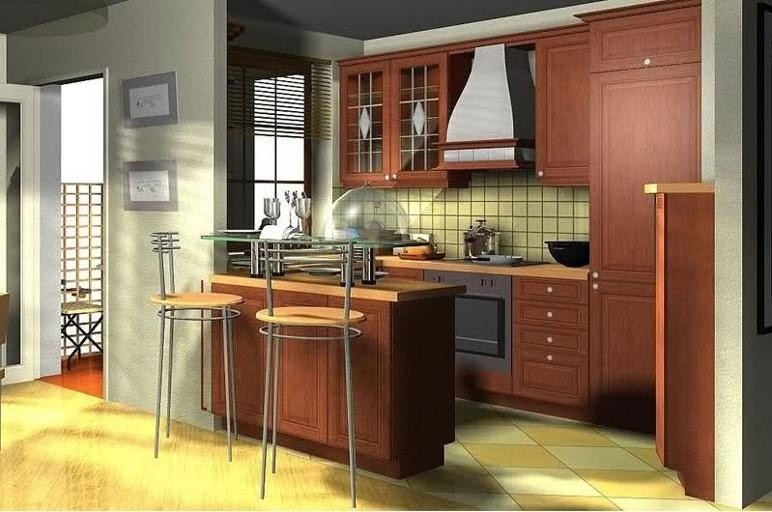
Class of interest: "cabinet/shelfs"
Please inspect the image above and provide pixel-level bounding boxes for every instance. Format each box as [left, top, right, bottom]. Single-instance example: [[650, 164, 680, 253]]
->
[[575, 3, 702, 434], [381, 267, 588, 423], [336, 49, 471, 188], [535, 29, 590, 187]]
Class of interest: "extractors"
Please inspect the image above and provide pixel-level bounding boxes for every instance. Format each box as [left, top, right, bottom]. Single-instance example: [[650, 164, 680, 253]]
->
[[442, 43, 537, 170]]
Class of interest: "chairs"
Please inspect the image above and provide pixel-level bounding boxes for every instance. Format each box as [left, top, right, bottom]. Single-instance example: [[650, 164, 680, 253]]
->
[[151, 232, 246, 462], [61, 279, 102, 371], [255, 241, 367, 509]]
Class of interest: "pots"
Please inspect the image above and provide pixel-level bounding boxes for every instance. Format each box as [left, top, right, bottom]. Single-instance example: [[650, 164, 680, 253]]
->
[[454, 254, 524, 266], [462, 219, 502, 259]]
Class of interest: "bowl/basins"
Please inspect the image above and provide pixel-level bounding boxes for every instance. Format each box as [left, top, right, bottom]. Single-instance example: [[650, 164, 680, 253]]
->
[[544, 241, 590, 267]]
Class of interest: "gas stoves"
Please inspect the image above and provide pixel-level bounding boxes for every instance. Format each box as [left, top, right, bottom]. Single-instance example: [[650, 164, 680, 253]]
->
[[441, 258, 552, 268]]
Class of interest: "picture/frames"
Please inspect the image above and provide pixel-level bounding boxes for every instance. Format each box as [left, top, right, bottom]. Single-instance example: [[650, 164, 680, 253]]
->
[[122, 71, 178, 130], [123, 160, 179, 211], [756, 2, 771, 333]]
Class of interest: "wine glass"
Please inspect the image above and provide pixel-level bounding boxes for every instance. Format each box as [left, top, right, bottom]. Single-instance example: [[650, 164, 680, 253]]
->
[[264, 189, 312, 241]]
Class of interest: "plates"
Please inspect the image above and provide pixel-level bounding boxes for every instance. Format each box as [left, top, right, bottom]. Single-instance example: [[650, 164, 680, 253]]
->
[[352, 270, 391, 277], [216, 229, 262, 233], [297, 267, 342, 276]]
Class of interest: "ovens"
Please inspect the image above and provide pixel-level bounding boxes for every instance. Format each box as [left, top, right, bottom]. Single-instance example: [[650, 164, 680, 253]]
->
[[423, 269, 513, 379]]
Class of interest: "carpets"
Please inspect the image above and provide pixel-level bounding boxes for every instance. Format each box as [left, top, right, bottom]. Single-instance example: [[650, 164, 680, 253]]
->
[[0, 379, 480, 511]]
